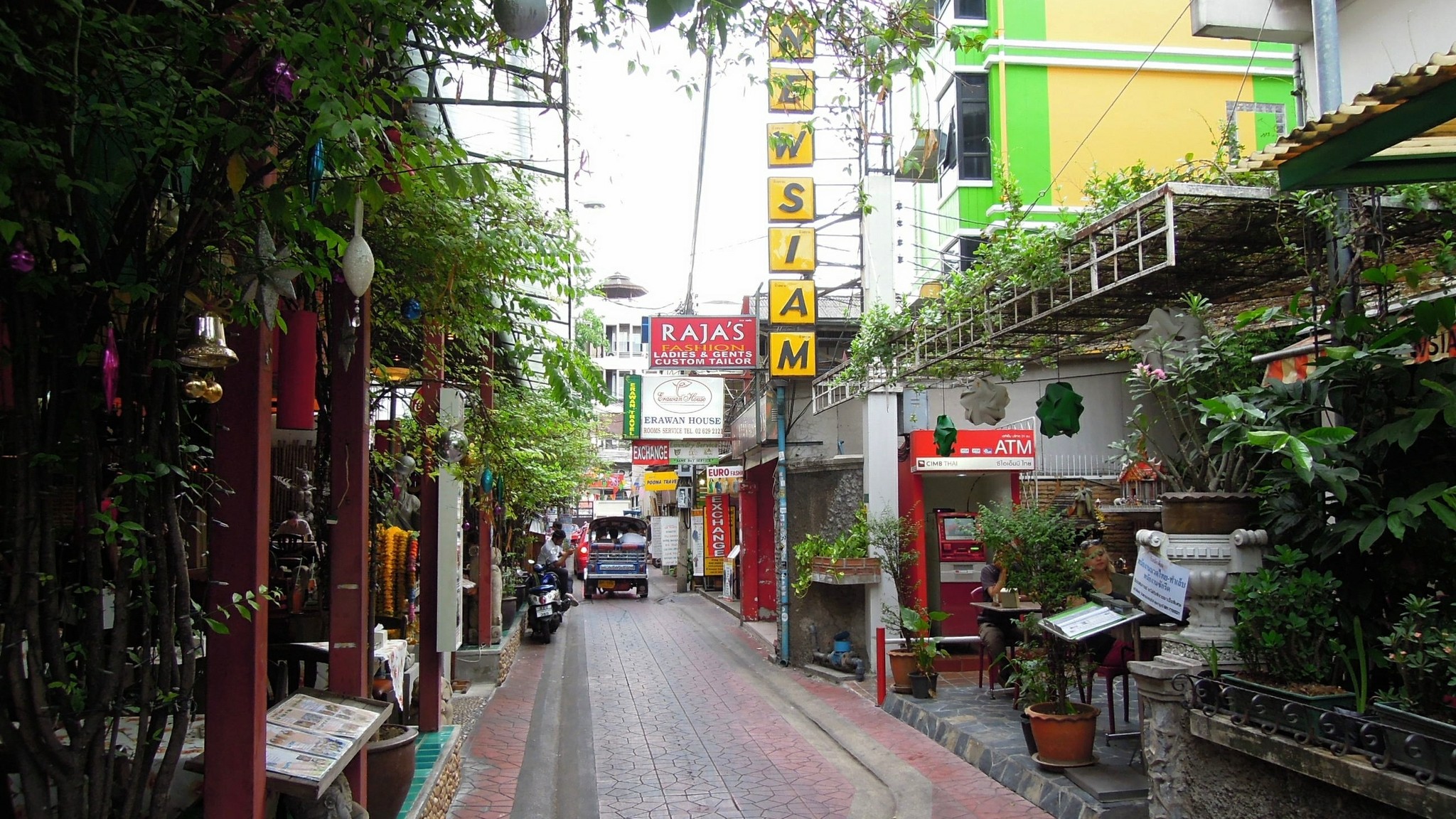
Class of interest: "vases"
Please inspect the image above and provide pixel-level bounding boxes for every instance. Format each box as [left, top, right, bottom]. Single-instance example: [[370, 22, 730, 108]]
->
[[1157, 493, 1267, 535], [366, 721, 419, 819]]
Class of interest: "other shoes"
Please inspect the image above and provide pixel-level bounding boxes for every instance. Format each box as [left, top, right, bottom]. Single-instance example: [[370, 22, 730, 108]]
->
[[565, 593, 578, 606]]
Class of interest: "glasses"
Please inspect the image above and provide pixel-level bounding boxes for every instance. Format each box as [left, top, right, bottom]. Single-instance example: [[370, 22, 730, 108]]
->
[[1080, 539, 1100, 549]]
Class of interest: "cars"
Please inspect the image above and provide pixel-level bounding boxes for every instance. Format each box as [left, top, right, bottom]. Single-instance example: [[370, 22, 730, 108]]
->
[[572, 528, 589, 579]]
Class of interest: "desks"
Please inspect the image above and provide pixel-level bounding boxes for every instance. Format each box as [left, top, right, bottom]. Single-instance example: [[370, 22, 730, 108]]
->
[[971, 602, 1063, 645], [287, 639, 408, 712], [271, 539, 328, 562]]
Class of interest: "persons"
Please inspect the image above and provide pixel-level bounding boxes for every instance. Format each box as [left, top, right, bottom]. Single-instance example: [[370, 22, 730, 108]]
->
[[709, 477, 714, 494], [375, 455, 422, 533], [724, 476, 731, 493], [977, 544, 1044, 704], [550, 521, 567, 570], [646, 514, 652, 563], [1064, 539, 1178, 677], [535, 528, 581, 607], [548, 526, 553, 533], [732, 476, 739, 493], [272, 509, 315, 549], [723, 565, 727, 598], [715, 476, 722, 495], [614, 523, 646, 545], [289, 463, 318, 523], [607, 529, 622, 544], [729, 571, 732, 601]]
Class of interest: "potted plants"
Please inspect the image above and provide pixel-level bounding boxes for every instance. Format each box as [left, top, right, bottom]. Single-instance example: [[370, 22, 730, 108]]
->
[[500, 528, 539, 630], [790, 493, 951, 698], [1219, 539, 1456, 787], [953, 497, 1106, 763]]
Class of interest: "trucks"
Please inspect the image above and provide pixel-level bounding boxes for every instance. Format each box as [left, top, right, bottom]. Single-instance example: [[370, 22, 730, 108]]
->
[[583, 516, 649, 599]]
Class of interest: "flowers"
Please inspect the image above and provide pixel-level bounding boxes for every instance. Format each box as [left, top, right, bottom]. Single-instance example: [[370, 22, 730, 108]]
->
[[1102, 288, 1291, 492]]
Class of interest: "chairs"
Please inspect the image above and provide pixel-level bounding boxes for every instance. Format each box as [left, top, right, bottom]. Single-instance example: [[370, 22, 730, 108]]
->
[[270, 532, 303, 623], [372, 615, 407, 722], [970, 587, 1132, 735]]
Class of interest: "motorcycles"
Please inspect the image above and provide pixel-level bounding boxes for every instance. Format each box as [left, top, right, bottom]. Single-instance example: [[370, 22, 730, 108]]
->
[[516, 555, 571, 645]]
